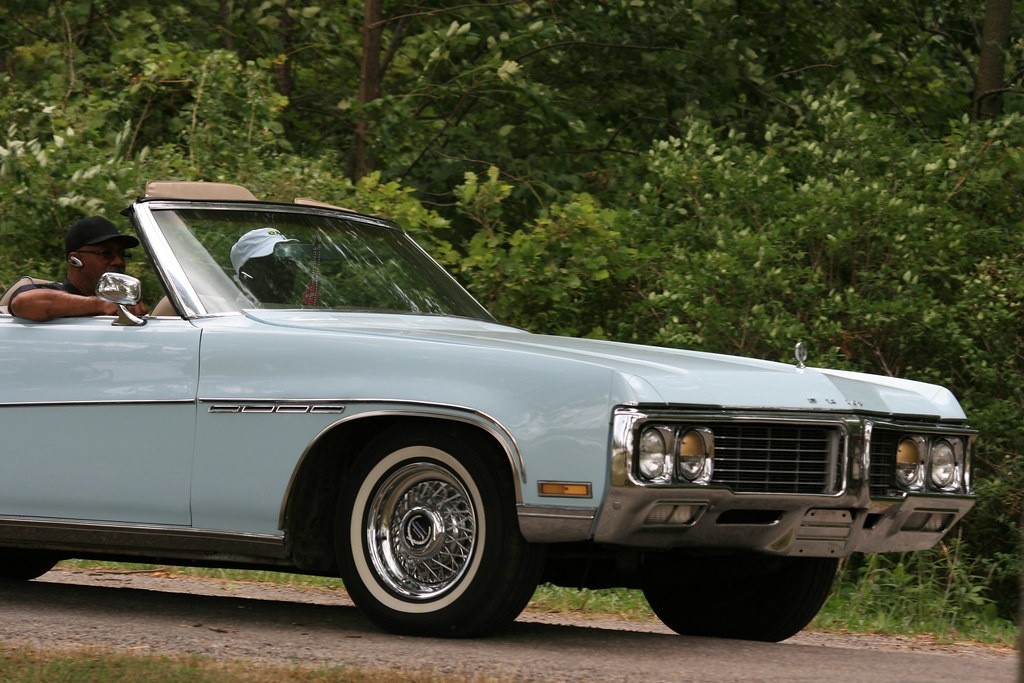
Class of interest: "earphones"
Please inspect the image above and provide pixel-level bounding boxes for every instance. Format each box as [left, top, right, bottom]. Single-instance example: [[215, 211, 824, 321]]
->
[[240, 271, 253, 281], [68, 256, 83, 267]]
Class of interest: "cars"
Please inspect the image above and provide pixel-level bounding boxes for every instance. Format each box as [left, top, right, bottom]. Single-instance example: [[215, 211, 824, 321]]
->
[[0, 182, 974, 643]]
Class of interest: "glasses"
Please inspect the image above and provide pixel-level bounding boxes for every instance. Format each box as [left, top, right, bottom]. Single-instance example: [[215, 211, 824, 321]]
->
[[74, 250, 133, 264]]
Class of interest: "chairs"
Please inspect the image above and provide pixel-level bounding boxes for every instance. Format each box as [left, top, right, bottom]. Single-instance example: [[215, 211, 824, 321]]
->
[[0, 276, 180, 317]]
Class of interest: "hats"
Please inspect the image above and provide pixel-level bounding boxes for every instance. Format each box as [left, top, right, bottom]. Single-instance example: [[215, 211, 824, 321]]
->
[[65, 216, 139, 263], [229, 227, 301, 277]]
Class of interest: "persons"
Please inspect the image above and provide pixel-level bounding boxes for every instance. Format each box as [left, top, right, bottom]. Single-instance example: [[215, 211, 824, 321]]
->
[[230, 227, 301, 304], [8, 217, 148, 322]]
